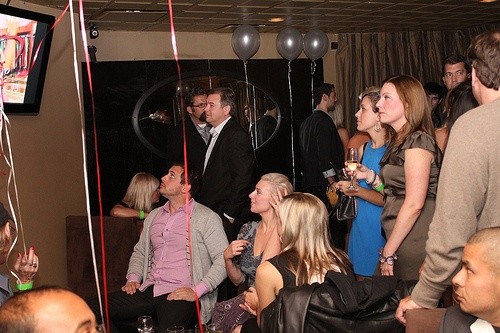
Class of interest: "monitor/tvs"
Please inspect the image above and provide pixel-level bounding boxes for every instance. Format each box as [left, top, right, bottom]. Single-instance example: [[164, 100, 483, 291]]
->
[[0, 4, 55, 115]]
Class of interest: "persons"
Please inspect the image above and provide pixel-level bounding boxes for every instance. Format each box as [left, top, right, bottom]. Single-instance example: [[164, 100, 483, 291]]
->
[[109, 172, 160, 218], [242, 104, 261, 132], [229, 191, 360, 333], [296, 81, 348, 253], [252, 99, 276, 149], [341, 73, 441, 282], [158, 110, 171, 125], [208, 172, 296, 333], [438, 226, 500, 333], [101, 162, 232, 333], [153, 26, 500, 254], [0, 284, 102, 333], [0, 203, 41, 308]]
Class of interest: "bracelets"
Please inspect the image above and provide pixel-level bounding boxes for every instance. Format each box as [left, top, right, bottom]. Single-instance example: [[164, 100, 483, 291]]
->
[[15, 279, 34, 291], [376, 182, 387, 193], [371, 176, 380, 186], [139, 210, 145, 220], [327, 179, 336, 189]]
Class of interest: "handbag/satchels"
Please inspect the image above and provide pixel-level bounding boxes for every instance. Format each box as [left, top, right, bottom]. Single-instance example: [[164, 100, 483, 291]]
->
[[329, 190, 357, 221]]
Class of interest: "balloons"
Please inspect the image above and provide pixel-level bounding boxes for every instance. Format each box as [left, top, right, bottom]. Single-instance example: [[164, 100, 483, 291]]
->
[[300, 28, 329, 62], [230, 26, 261, 63], [276, 26, 306, 63]]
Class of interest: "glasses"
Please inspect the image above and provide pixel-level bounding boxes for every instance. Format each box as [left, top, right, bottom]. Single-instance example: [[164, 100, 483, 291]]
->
[[9, 225, 16, 233], [192, 103, 208, 108]]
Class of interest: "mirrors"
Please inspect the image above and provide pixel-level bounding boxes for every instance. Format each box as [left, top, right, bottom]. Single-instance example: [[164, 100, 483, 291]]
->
[[132, 69, 284, 163]]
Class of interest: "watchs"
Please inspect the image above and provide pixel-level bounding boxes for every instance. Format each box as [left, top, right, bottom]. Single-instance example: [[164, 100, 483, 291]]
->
[[365, 172, 377, 186]]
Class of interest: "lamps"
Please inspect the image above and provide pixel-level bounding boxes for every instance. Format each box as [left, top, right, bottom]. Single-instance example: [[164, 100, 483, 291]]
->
[[90, 25, 99, 39]]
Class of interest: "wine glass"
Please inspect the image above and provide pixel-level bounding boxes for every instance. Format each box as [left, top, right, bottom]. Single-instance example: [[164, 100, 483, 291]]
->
[[345, 147, 358, 193]]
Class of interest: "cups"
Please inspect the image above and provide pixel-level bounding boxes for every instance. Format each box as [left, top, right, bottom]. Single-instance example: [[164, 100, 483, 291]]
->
[[195, 323, 223, 333], [166, 326, 184, 333], [137, 316, 154, 333]]
